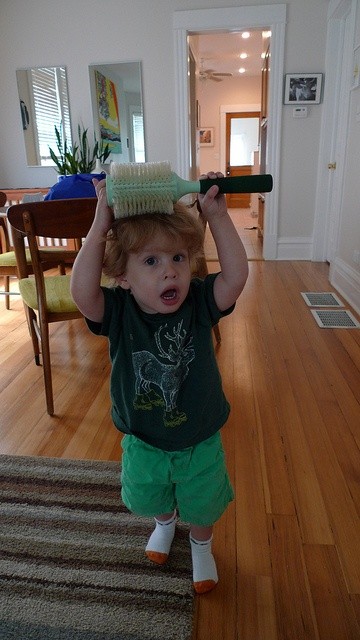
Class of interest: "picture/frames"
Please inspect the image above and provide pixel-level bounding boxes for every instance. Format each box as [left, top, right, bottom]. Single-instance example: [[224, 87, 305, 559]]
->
[[95, 70, 123, 155], [283, 73, 323, 106], [198, 127, 213, 147]]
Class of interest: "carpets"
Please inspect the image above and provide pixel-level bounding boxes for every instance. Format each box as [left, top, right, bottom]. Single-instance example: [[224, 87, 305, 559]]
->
[[0, 453, 195, 638], [311, 309, 358, 328], [301, 291, 344, 308]]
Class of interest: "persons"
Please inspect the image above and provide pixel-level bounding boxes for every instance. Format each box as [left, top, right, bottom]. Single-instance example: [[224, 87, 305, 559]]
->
[[70, 172, 249, 594]]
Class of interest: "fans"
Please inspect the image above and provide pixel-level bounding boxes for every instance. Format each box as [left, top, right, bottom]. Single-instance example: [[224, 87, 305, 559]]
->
[[200, 69, 232, 84]]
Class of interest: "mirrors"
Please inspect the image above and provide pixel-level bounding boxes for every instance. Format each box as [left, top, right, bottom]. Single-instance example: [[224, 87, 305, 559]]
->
[[88, 62, 147, 165], [15, 66, 75, 168]]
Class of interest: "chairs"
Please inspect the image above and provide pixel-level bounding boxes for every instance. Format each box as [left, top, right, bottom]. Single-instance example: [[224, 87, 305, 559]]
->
[[7, 198, 125, 414], [1, 214, 58, 311]]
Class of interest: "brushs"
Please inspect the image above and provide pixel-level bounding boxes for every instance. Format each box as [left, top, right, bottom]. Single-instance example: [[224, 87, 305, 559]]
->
[[105, 160, 275, 219]]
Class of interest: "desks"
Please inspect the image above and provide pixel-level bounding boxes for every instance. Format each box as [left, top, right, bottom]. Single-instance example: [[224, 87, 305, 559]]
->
[[1, 202, 223, 346]]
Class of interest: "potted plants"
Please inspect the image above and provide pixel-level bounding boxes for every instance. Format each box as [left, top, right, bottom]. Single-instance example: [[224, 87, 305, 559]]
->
[[79, 117, 108, 175]]
